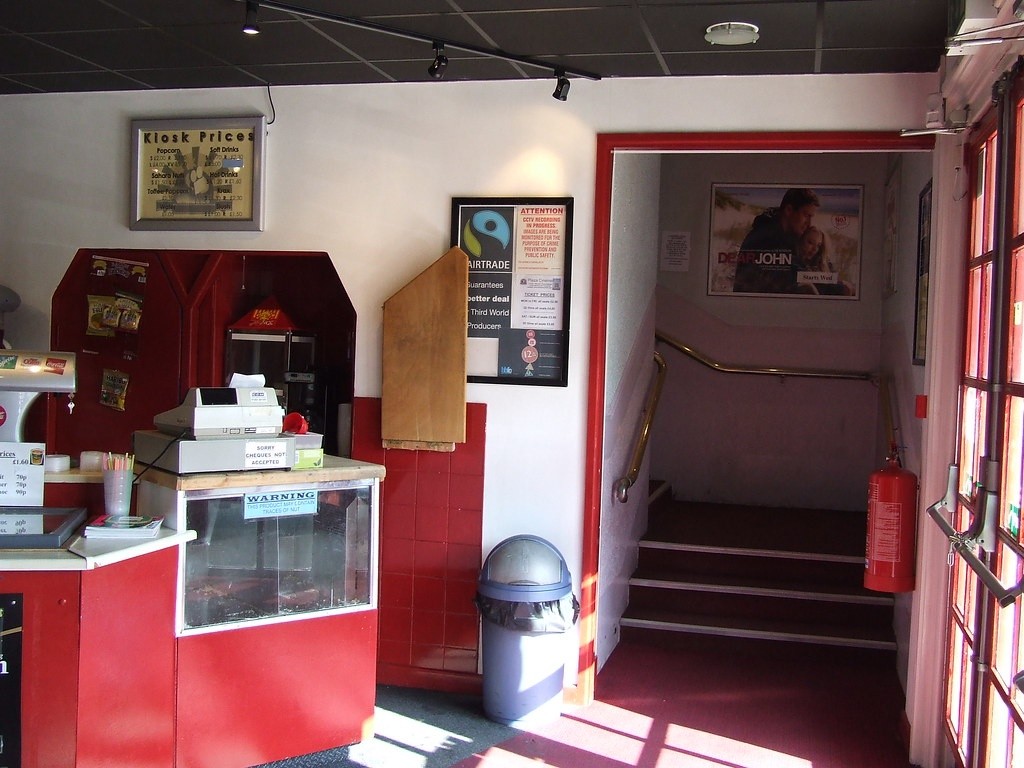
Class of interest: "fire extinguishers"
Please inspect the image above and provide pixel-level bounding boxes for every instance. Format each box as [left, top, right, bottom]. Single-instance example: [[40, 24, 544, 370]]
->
[[861, 437, 920, 593]]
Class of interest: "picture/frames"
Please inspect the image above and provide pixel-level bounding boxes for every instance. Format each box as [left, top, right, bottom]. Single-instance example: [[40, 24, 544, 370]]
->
[[450, 196, 574, 388], [129, 114, 266, 232], [706, 182, 865, 302]]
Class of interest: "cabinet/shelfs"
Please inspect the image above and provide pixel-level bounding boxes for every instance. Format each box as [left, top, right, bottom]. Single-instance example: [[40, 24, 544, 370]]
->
[[0, 523, 194, 768], [137, 454, 387, 768]]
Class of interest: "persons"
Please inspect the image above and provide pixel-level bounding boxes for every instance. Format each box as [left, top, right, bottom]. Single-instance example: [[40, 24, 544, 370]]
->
[[733, 188, 856, 296]]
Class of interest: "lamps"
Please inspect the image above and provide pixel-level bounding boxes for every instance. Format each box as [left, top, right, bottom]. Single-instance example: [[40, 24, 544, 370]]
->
[[241, 0, 602, 102]]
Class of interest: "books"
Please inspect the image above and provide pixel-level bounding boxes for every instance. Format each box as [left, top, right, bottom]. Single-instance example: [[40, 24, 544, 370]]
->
[[84, 514, 165, 539]]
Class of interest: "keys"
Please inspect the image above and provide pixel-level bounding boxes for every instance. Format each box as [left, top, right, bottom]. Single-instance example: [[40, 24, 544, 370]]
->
[[68, 392, 75, 414]]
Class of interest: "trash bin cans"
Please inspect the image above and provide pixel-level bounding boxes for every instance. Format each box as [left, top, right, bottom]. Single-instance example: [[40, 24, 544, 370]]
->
[[472, 533, 578, 731]]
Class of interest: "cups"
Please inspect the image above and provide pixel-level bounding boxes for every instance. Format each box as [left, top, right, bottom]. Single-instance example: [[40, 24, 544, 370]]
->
[[103, 469, 133, 516]]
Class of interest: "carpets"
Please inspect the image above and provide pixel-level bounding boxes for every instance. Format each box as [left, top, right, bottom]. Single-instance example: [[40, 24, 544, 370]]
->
[[349, 683, 529, 768]]
